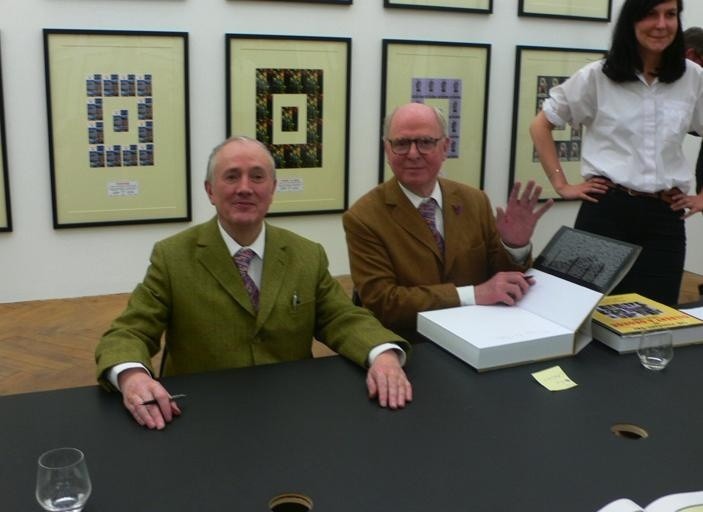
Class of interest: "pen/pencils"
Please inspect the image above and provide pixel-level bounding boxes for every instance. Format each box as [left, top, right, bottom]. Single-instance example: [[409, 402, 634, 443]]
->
[[292, 292, 297, 305], [139, 394, 188, 405], [523, 276, 533, 280]]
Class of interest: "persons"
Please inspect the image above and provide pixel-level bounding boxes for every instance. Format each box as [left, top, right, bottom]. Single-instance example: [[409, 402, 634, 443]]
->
[[93, 135, 414, 431], [343, 102, 555, 345], [683, 27, 703, 194], [528, 0, 703, 307]]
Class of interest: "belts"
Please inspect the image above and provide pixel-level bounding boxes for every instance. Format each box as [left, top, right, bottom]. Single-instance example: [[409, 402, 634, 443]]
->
[[606, 183, 664, 198]]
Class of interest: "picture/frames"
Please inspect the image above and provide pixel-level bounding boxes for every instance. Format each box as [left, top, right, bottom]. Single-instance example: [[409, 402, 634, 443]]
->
[[384, 0, 493, 13], [378, 38, 491, 191], [518, 0, 612, 22], [225, 33, 352, 216], [43, 28, 192, 229], [0, 52, 13, 232], [228, 0, 353, 4], [507, 45, 609, 203]]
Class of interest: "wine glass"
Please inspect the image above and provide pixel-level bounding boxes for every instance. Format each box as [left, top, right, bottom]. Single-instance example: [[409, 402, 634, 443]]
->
[[35, 447, 92, 512]]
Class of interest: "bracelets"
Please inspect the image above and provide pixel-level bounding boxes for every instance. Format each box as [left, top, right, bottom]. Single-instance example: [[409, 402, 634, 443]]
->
[[548, 168, 561, 179]]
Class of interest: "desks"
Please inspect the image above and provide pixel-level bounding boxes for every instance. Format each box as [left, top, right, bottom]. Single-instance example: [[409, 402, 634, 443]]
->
[[0, 300, 703, 511]]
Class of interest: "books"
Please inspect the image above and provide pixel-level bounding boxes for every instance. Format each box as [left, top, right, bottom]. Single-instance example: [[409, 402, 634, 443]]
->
[[415, 225, 645, 374], [591, 292, 702, 355]]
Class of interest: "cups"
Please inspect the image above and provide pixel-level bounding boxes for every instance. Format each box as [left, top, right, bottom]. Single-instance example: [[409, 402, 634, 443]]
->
[[637, 332, 674, 371]]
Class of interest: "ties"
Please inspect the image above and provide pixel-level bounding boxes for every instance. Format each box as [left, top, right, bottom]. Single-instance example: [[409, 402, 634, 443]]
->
[[232, 247, 261, 314], [417, 200, 446, 258]]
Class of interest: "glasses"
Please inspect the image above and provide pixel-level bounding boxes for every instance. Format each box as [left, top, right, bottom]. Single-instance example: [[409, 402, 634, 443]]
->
[[384, 136, 448, 157]]
